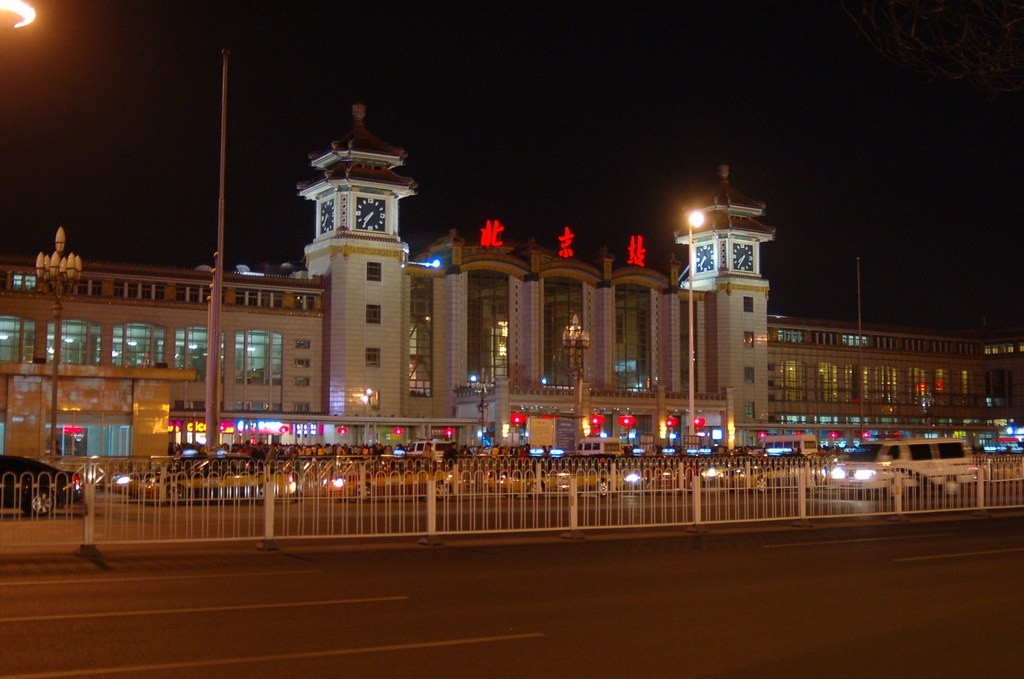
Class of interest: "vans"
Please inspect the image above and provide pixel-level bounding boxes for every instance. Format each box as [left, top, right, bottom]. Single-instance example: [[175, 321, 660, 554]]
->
[[405, 439, 453, 456], [576, 436, 622, 456], [830, 436, 979, 499]]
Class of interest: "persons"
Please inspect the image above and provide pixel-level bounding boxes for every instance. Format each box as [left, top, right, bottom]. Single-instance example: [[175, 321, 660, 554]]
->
[[165, 439, 843, 478]]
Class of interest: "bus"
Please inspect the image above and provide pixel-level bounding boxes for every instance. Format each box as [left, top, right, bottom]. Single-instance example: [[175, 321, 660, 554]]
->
[[758, 432, 818, 456]]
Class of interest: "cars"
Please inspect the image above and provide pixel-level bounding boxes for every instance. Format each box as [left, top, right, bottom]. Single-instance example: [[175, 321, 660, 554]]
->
[[0, 453, 82, 517], [113, 445, 830, 505]]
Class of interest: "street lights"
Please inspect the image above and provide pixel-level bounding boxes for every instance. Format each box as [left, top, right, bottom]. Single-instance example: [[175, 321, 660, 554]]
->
[[685, 209, 704, 436], [35, 225, 82, 457]]
